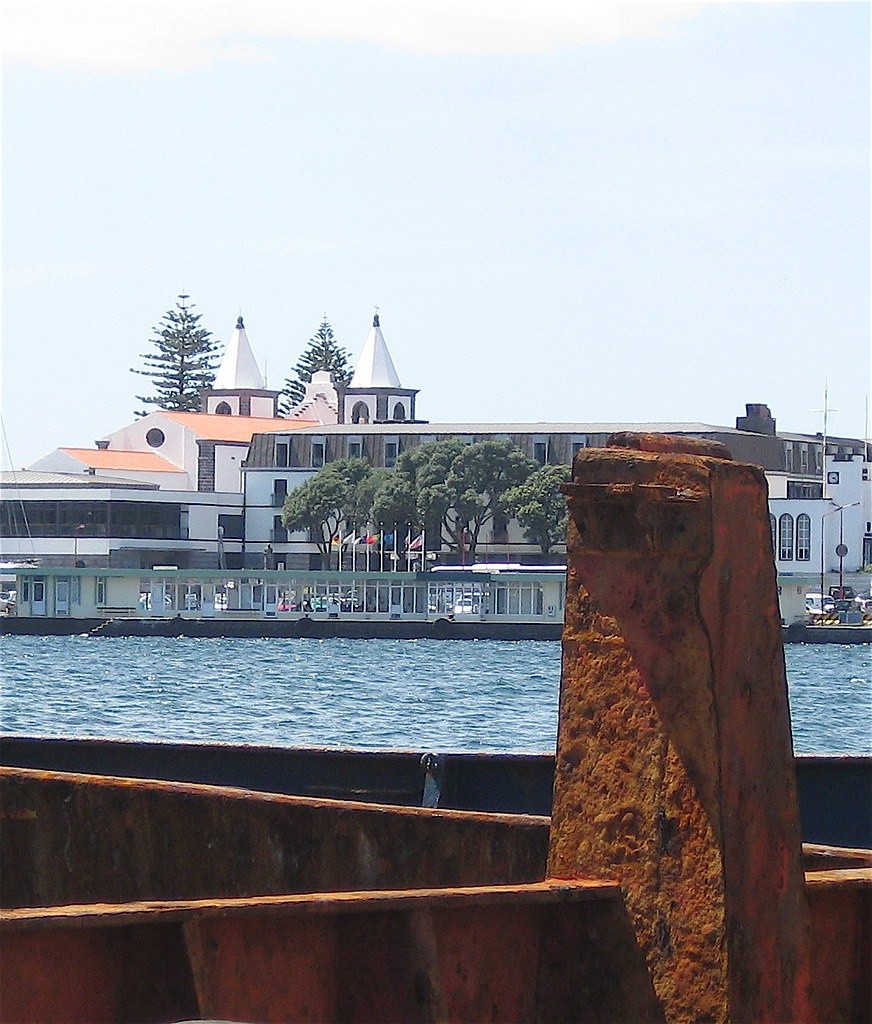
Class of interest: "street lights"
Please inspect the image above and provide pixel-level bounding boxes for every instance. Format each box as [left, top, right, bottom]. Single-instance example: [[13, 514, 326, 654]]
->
[[829, 500, 862, 626], [821, 507, 843, 612]]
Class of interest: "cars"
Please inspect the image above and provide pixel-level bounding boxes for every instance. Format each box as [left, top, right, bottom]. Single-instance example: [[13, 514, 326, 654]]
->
[[429, 592, 489, 614], [278, 590, 360, 612], [807, 584, 872, 624]]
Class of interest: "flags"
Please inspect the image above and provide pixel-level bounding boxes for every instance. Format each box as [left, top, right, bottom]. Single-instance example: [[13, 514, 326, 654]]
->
[[342, 533, 353, 544], [384, 534, 394, 545], [331, 532, 340, 546], [403, 536, 409, 548], [410, 536, 421, 550], [354, 535, 367, 547], [368, 533, 381, 545]]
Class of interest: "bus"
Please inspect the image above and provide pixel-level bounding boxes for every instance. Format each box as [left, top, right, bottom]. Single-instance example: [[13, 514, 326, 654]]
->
[[431, 563, 567, 590]]
